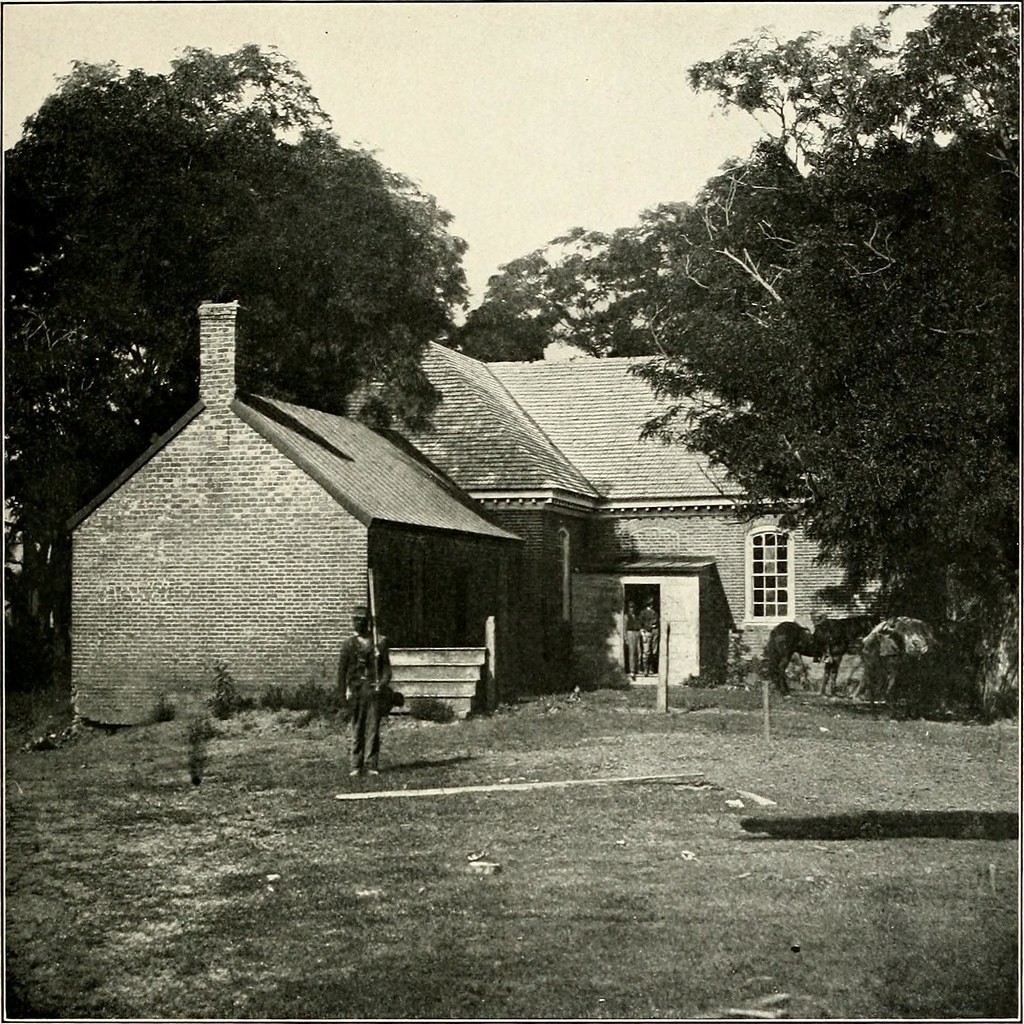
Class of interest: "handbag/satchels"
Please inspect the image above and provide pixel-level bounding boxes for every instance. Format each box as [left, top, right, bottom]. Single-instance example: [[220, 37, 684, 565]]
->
[[377, 684, 404, 717]]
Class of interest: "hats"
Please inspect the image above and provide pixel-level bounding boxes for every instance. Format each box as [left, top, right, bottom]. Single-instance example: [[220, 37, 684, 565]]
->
[[643, 596, 653, 604], [626, 601, 638, 609], [351, 607, 372, 617]]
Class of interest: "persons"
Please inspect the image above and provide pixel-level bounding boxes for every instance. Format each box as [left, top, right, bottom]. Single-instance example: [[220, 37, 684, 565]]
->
[[623, 597, 659, 678], [818, 619, 845, 696], [765, 590, 782, 616], [337, 607, 392, 777], [887, 645, 904, 720]]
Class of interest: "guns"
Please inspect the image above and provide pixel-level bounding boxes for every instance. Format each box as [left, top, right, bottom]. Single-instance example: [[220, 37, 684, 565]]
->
[[366, 569, 383, 692]]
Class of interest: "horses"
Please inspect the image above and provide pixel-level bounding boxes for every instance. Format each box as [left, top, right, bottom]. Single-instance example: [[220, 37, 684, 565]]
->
[[767, 611, 882, 702]]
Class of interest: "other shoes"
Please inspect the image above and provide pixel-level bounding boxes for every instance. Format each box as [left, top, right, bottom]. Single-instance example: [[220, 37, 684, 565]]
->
[[367, 769, 379, 776], [349, 769, 360, 777], [644, 668, 648, 677], [630, 672, 634, 679]]
